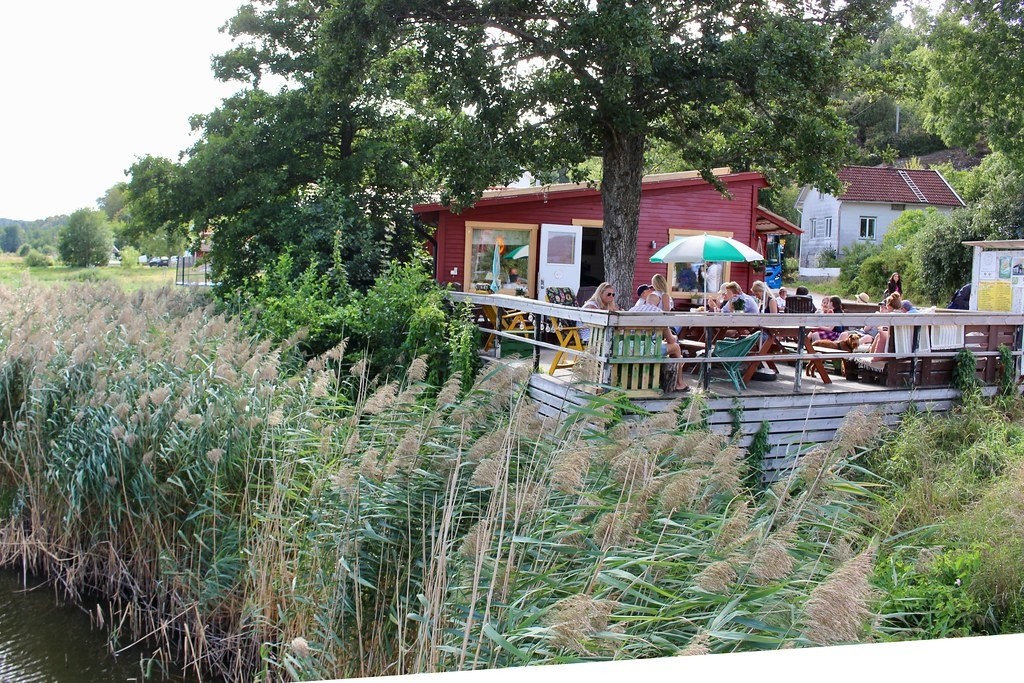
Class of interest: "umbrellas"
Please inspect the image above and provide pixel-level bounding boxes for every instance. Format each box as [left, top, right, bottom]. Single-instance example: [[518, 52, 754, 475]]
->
[[489, 242, 501, 294], [648, 233, 765, 334], [504, 244, 529, 259]]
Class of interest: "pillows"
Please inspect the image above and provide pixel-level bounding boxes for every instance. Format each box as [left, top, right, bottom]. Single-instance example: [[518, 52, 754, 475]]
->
[[870, 331, 887, 362]]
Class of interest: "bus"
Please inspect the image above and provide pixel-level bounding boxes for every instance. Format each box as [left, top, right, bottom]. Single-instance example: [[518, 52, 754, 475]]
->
[[764, 233, 788, 289]]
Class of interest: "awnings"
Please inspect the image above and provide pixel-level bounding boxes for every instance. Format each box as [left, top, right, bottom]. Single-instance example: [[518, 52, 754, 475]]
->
[[756, 204, 805, 236]]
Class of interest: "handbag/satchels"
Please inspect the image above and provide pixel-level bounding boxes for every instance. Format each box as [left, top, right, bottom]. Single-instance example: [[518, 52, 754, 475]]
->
[[884, 290, 891, 299]]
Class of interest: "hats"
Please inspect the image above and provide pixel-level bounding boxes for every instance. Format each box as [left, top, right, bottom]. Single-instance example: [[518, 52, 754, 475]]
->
[[636, 283, 653, 296], [854, 293, 870, 305], [718, 282, 731, 293], [878, 299, 887, 306], [901, 300, 912, 311]]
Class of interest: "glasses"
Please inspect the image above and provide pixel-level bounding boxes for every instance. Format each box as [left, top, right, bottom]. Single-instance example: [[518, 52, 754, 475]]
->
[[604, 292, 616, 297]]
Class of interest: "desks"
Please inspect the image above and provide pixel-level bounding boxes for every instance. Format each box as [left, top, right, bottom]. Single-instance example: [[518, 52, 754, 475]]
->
[[679, 339, 716, 374], [680, 302, 709, 307], [779, 342, 848, 378]]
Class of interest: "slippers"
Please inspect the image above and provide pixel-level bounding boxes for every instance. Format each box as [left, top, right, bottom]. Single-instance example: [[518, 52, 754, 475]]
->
[[676, 385, 691, 392]]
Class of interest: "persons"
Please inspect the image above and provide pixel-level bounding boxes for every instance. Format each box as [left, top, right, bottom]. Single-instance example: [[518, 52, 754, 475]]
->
[[796, 286, 816, 341], [679, 263, 698, 293], [887, 272, 903, 295], [626, 274, 687, 390], [510, 269, 520, 295], [709, 278, 778, 371], [577, 282, 619, 382], [698, 262, 722, 292], [837, 292, 916, 362], [947, 283, 971, 310], [807, 297, 845, 342], [775, 287, 794, 341]]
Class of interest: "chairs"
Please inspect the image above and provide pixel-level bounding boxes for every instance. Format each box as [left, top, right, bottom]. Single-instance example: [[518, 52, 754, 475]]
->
[[697, 330, 762, 394], [783, 296, 817, 313], [543, 287, 587, 375], [474, 283, 534, 352]]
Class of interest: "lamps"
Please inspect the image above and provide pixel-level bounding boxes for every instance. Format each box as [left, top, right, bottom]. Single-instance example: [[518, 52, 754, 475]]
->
[[652, 240, 656, 248]]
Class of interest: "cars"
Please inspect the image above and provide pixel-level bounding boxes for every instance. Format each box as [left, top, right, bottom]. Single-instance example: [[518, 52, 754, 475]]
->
[[149, 256, 169, 267]]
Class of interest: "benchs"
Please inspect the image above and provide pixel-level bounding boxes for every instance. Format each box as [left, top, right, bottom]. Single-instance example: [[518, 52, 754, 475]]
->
[[846, 325, 1017, 386], [842, 302, 880, 315]]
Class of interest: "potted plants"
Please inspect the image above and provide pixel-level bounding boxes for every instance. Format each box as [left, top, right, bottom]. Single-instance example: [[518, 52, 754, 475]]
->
[[753, 260, 767, 273], [690, 292, 700, 304]]
